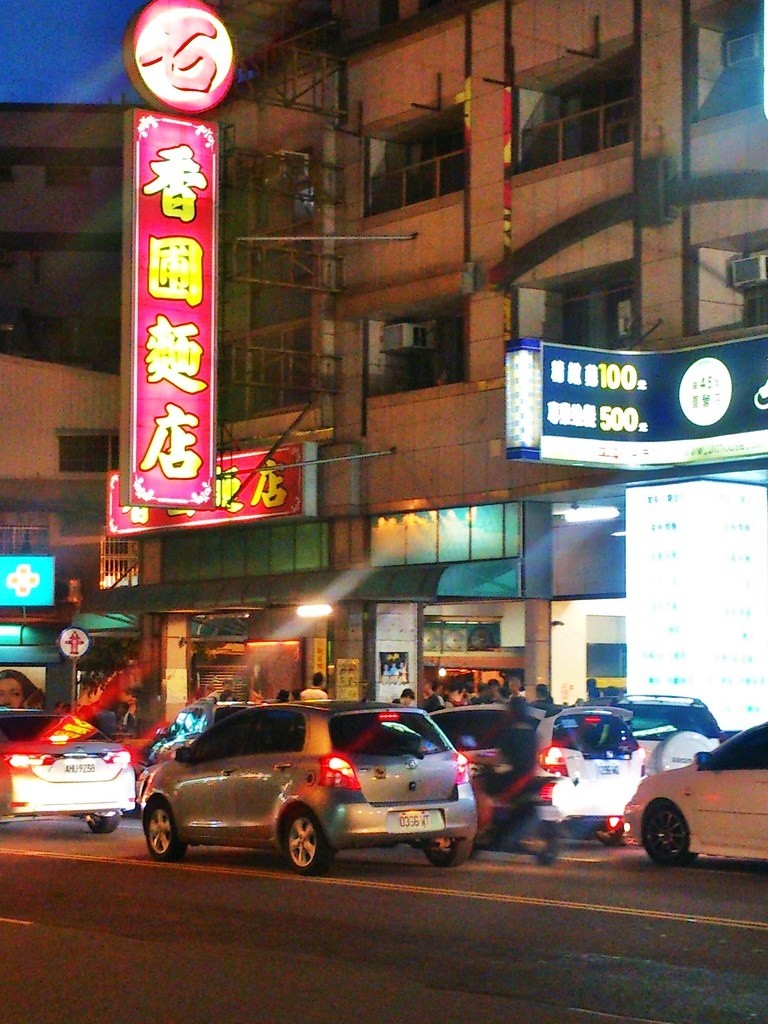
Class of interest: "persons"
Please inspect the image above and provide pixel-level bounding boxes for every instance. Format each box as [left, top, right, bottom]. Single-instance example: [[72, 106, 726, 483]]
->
[[0, 669, 45, 709], [191, 653, 198, 688], [587, 678, 600, 699], [115, 702, 134, 732], [392, 673, 553, 716], [206, 666, 328, 703], [340, 669, 353, 686], [55, 701, 81, 716], [382, 661, 405, 677]]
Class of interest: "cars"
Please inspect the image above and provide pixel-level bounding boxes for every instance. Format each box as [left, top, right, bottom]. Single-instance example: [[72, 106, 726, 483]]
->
[[139, 699, 478, 876], [621, 717, 768, 869]]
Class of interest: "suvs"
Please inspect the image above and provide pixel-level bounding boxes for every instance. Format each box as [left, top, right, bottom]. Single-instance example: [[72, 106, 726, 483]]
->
[[404, 694, 724, 850], [145, 697, 269, 766]]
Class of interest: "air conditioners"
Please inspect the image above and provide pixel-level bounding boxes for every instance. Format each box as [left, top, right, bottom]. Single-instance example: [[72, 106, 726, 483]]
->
[[617, 301, 632, 339], [604, 117, 635, 148], [265, 152, 311, 187], [725, 31, 764, 72], [381, 323, 436, 352], [729, 255, 767, 286]]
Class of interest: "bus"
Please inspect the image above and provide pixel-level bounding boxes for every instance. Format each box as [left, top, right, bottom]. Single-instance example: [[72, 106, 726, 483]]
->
[[0, 706, 137, 834]]
